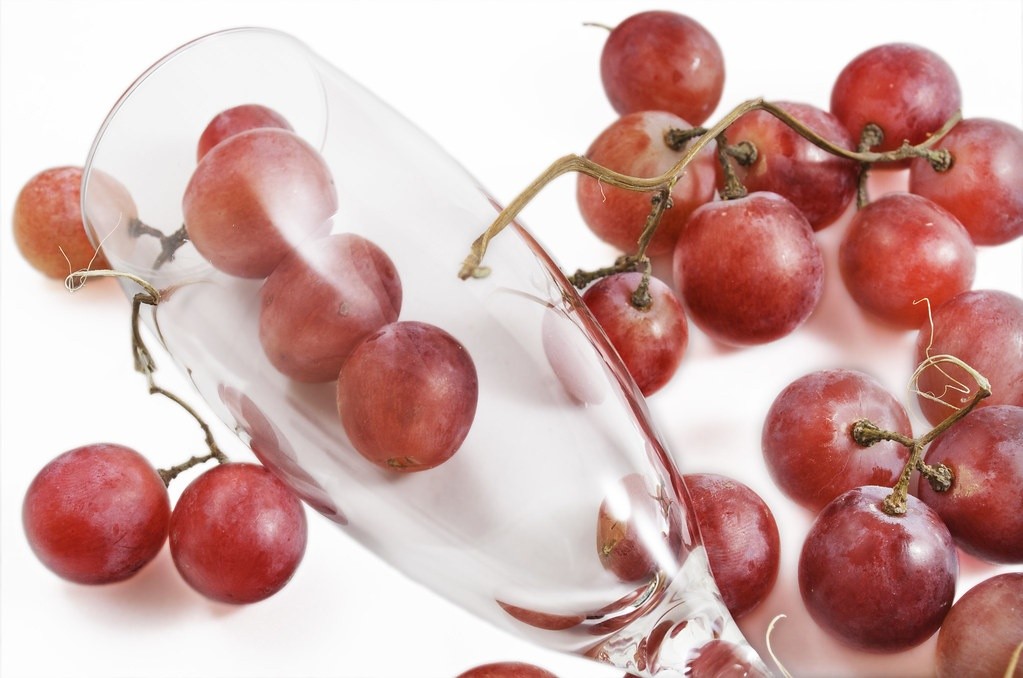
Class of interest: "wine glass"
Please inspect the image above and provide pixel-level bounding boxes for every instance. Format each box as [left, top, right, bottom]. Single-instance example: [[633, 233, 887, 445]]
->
[[76, 24, 773, 678]]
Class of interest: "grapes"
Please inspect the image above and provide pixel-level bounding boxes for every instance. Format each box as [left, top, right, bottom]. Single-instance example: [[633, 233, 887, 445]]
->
[[9, 104, 474, 607], [457, 10, 1023, 678]]
